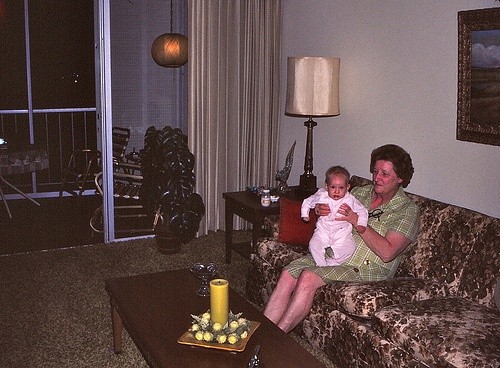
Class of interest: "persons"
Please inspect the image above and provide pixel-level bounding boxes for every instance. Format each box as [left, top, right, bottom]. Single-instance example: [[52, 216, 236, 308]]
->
[[300, 166, 369, 268], [261, 144, 420, 334]]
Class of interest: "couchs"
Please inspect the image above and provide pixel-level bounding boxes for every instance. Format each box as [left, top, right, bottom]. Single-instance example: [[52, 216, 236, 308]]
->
[[245, 174, 500, 368]]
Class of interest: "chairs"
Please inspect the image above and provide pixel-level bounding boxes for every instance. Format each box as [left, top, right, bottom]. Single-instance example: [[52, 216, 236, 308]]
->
[[59, 127, 158, 233]]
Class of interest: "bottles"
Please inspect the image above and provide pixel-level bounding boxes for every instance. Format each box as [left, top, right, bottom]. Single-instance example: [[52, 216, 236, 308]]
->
[[260, 189, 271, 207]]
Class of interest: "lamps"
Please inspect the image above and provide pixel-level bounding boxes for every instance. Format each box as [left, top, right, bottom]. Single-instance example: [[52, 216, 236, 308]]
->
[[152, 1, 188, 68], [285, 56, 341, 193]]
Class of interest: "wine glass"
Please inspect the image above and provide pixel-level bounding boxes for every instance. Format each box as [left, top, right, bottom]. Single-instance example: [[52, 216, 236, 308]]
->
[[190, 262, 217, 296]]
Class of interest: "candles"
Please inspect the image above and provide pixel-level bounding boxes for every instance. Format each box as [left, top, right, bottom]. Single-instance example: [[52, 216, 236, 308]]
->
[[209, 278, 229, 325]]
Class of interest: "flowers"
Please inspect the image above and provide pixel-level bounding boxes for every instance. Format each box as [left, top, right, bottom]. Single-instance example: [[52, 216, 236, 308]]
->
[[189, 309, 248, 344]]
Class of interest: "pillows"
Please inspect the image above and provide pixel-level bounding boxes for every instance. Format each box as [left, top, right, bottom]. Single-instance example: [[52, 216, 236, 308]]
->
[[278, 196, 319, 245]]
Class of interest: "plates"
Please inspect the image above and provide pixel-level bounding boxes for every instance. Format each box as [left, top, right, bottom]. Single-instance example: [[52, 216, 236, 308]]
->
[[177, 317, 261, 352]]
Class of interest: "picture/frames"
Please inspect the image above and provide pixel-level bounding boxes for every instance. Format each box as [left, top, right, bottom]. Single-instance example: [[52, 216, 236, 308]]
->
[[457, 7, 500, 147]]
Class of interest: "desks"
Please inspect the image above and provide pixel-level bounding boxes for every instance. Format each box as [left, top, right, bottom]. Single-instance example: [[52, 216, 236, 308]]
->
[[0, 144, 49, 219]]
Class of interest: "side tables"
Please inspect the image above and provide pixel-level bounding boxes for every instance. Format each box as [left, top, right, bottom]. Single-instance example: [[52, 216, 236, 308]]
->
[[223, 187, 298, 264]]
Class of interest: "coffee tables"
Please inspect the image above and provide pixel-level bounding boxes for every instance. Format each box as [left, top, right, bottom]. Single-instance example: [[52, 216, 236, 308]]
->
[[105, 269, 328, 368]]
